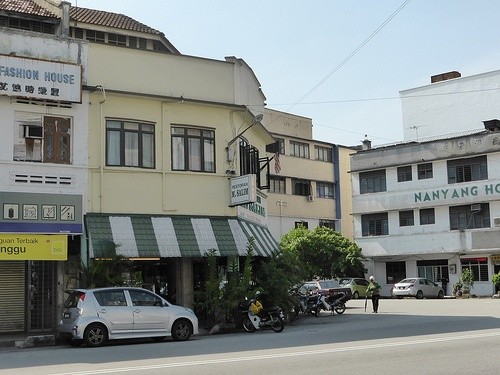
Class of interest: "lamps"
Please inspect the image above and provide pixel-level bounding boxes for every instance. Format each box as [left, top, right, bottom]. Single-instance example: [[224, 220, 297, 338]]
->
[[226, 115, 264, 161]]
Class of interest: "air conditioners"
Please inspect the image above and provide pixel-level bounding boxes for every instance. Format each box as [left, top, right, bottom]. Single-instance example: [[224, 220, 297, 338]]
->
[[471, 204, 481, 211], [493, 217, 500, 227], [307, 195, 315, 201], [26, 126, 43, 140]]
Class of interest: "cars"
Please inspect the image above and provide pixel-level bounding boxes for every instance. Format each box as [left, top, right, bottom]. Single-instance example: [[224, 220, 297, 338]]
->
[[57, 286, 199, 348], [302, 279, 353, 303], [338, 277, 374, 299], [392, 277, 445, 299]]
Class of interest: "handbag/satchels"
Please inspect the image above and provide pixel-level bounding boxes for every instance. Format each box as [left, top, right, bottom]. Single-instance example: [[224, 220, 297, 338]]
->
[[373, 287, 381, 294]]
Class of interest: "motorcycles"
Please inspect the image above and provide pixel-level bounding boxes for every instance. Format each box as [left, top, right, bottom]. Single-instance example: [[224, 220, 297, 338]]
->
[[239, 290, 286, 334], [290, 286, 346, 319]]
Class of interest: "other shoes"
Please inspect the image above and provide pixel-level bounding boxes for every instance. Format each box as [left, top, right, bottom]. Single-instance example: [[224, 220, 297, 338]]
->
[[259, 326, 264, 331]]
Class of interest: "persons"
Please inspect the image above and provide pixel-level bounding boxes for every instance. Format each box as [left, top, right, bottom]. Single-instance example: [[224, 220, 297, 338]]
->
[[366, 275, 382, 313], [441, 276, 448, 295]]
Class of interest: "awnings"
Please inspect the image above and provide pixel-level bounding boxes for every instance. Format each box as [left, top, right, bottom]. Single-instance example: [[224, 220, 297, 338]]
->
[[86, 213, 284, 258]]
